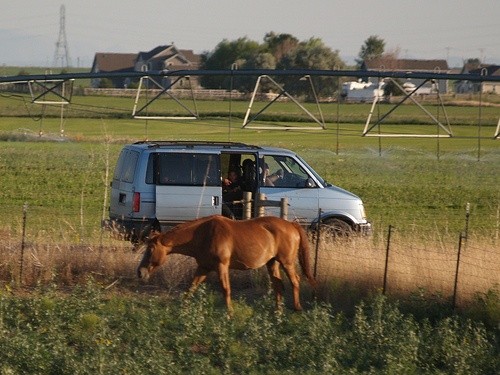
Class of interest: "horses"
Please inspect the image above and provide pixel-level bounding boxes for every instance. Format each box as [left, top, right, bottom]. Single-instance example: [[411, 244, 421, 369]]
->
[[136, 214, 314, 316]]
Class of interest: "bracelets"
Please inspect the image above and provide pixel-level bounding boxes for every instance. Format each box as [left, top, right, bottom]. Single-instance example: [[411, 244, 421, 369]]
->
[[275, 174, 280, 179]]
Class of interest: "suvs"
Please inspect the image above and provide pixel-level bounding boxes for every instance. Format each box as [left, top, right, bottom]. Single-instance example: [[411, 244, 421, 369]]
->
[[109, 139, 371, 244]]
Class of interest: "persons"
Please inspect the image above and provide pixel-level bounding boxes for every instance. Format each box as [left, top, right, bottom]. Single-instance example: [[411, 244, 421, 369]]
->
[[221, 159, 284, 221]]
